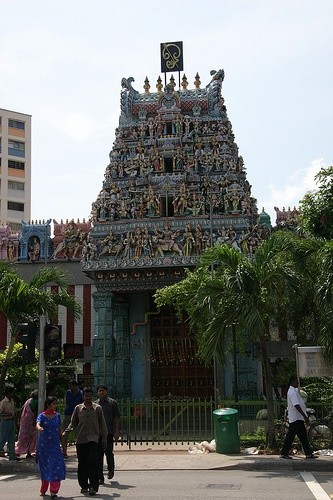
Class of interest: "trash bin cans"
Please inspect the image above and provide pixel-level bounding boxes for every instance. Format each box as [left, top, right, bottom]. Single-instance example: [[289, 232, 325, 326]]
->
[[213, 408, 241, 453]]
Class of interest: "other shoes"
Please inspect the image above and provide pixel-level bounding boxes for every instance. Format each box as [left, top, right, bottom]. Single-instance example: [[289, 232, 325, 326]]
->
[[306, 454, 318, 458], [99, 479, 104, 484], [40, 493, 45, 496], [280, 457, 292, 459], [89, 487, 96, 496], [51, 493, 57, 497], [108, 470, 114, 479], [64, 454, 68, 457], [80, 488, 88, 493], [26, 454, 33, 458], [9, 458, 20, 461]]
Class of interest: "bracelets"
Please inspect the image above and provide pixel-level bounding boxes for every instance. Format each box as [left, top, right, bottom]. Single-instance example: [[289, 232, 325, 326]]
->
[[39, 427, 42, 431]]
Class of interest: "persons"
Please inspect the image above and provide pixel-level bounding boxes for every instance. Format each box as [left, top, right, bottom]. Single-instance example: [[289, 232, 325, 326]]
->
[[61, 387, 108, 497], [8, 220, 270, 263], [34, 396, 68, 498], [0, 386, 20, 462], [57, 379, 85, 459], [94, 384, 122, 484], [279, 376, 321, 460], [89, 118, 260, 221], [13, 389, 39, 459]]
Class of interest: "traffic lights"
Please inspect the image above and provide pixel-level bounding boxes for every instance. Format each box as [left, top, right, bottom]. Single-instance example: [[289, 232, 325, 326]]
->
[[44, 324, 62, 360], [19, 323, 36, 360]]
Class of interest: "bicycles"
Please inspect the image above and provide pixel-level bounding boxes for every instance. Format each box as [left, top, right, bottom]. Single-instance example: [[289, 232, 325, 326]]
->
[[266, 405, 333, 451]]
